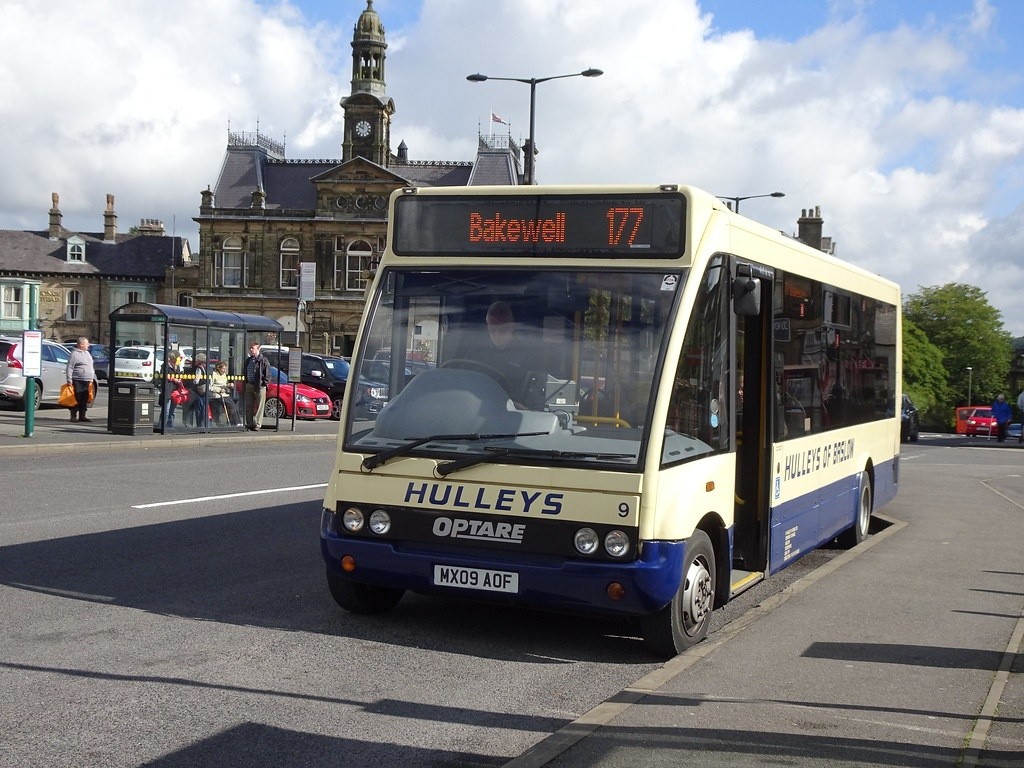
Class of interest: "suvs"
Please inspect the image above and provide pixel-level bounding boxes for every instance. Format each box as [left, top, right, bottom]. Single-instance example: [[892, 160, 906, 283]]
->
[[0, 337, 98, 411], [259, 348, 389, 421]]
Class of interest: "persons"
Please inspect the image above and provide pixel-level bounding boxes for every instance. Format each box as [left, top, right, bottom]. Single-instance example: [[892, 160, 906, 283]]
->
[[466, 301, 542, 409], [67, 336, 95, 423], [243, 342, 272, 430], [158, 349, 182, 428], [192, 354, 207, 429], [1017, 389, 1024, 443], [991, 394, 1012, 443], [208, 362, 243, 428]]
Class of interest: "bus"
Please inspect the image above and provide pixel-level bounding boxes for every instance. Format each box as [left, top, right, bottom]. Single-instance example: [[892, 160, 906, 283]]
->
[[322, 181, 904, 662]]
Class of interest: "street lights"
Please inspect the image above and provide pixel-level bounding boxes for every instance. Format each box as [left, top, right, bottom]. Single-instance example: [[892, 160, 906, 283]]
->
[[464, 69, 603, 188]]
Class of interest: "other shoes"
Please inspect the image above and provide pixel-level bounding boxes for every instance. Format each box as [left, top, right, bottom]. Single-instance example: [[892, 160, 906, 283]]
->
[[245, 425, 261, 432]]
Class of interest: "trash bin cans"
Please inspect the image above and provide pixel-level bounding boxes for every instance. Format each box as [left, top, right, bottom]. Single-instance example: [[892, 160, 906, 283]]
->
[[111, 382, 155, 436]]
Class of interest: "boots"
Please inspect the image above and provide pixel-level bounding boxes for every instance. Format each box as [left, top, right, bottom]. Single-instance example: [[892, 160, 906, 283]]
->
[[70, 410, 78, 422], [79, 410, 93, 422]]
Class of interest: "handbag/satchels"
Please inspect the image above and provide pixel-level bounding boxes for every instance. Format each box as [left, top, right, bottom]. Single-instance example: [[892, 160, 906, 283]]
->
[[58, 385, 78, 407], [86, 383, 93, 403]]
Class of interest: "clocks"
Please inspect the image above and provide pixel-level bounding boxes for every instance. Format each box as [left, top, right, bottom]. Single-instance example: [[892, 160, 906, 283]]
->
[[354, 119, 373, 138]]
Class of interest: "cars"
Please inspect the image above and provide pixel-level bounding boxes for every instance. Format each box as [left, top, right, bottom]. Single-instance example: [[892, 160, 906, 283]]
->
[[966, 407, 1000, 438], [263, 365, 332, 420], [1006, 424, 1022, 437], [106, 347, 170, 384]]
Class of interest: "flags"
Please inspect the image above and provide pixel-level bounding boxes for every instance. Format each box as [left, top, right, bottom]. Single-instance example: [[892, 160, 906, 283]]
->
[[492, 114, 508, 125]]
